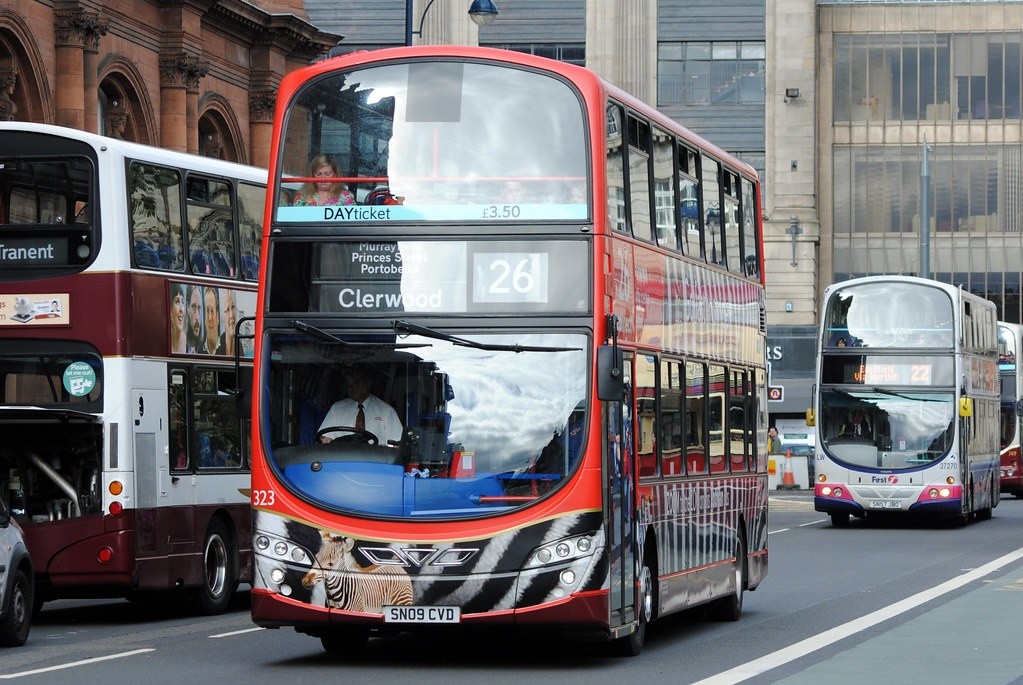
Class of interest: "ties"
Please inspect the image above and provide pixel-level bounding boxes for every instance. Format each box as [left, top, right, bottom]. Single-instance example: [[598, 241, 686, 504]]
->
[[356, 403, 365, 435]]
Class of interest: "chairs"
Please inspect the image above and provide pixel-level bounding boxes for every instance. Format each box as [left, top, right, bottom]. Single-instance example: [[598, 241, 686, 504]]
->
[[136, 242, 260, 281], [959, 218, 974, 231], [195, 431, 212, 467]]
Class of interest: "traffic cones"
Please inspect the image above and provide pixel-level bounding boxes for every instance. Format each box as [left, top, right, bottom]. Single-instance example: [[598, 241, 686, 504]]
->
[[775, 448, 800, 491]]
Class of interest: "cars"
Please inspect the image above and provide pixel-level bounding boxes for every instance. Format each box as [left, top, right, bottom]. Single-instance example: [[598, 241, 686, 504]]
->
[[0, 498, 34, 647]]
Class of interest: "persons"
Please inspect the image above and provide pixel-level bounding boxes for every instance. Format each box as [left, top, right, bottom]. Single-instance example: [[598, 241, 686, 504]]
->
[[843, 409, 869, 438], [501, 172, 585, 205], [203, 286, 223, 355], [316, 364, 403, 447], [293, 154, 356, 206], [219, 289, 244, 356], [169, 283, 186, 353], [836, 337, 847, 348], [186, 285, 203, 353], [767, 428, 781, 452]]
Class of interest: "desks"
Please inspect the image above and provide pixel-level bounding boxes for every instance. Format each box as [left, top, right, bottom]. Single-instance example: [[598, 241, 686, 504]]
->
[[991, 105, 1013, 119]]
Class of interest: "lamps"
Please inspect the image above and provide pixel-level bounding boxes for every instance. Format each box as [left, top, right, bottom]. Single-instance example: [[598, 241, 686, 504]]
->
[[784, 88, 800, 103]]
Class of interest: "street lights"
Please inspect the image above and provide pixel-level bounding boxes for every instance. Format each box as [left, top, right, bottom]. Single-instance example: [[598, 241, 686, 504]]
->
[[404, 0, 500, 48]]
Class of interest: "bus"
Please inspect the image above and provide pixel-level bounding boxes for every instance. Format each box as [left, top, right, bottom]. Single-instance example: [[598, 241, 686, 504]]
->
[[235, 44, 768, 660], [0, 121, 375, 617], [997, 320, 1023, 500], [805, 274, 1001, 527]]
